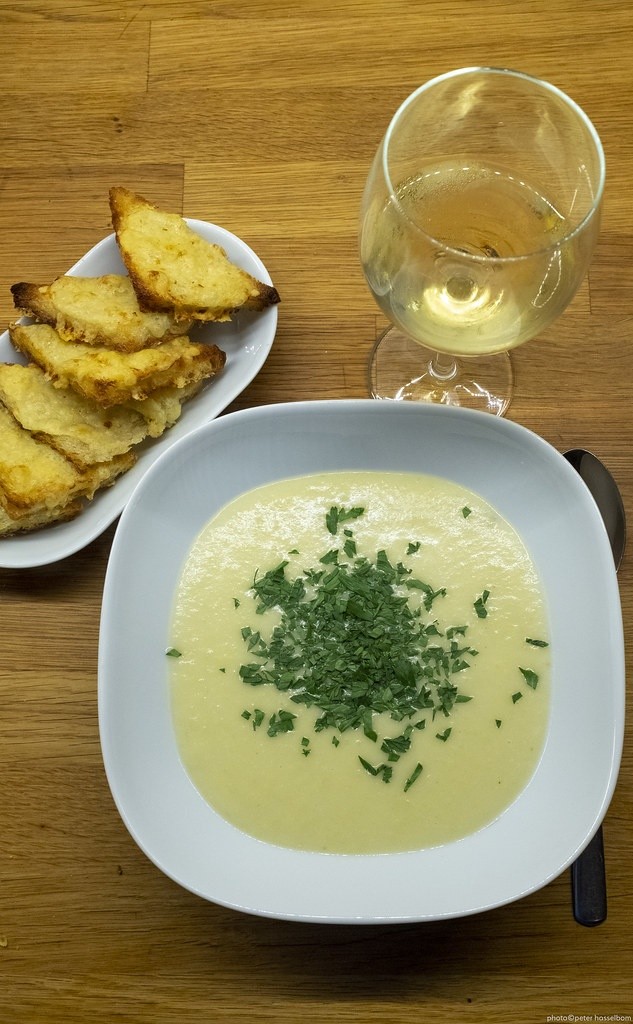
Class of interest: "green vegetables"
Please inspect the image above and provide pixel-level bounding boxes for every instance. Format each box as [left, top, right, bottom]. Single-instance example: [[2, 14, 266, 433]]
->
[[164, 504, 551, 792]]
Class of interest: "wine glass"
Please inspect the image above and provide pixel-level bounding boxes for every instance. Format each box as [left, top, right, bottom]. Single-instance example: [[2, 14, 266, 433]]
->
[[354, 65, 607, 417]]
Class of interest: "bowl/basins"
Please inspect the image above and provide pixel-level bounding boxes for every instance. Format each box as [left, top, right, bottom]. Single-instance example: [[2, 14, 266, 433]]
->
[[96, 398, 626, 927]]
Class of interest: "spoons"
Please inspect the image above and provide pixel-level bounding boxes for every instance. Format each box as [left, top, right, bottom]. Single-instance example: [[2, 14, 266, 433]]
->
[[556, 448, 627, 928]]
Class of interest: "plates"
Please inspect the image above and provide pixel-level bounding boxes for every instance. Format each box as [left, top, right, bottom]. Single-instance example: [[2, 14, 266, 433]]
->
[[0, 213, 278, 570]]
